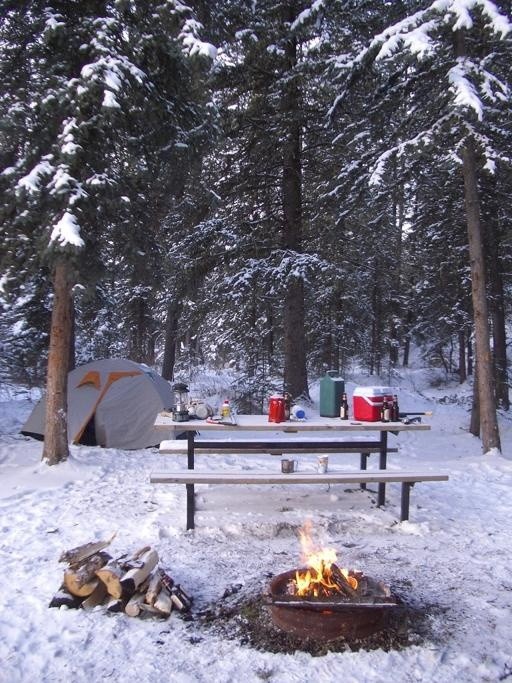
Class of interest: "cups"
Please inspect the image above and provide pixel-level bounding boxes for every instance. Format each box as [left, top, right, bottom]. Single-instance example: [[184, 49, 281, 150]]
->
[[268, 390, 290, 423], [317, 454, 329, 473], [188, 400, 219, 419], [281, 459, 298, 473]]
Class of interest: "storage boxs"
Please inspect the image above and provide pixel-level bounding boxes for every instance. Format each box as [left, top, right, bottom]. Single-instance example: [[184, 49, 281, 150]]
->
[[353, 385, 399, 421]]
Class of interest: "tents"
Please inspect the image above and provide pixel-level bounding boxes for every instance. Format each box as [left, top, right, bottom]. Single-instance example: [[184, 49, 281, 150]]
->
[[17, 356, 197, 452]]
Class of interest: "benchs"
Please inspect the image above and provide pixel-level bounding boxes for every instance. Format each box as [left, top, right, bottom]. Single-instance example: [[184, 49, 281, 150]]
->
[[150, 436, 449, 531]]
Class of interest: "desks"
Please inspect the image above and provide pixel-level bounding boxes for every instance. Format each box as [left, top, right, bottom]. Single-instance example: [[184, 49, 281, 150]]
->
[[155, 414, 432, 510]]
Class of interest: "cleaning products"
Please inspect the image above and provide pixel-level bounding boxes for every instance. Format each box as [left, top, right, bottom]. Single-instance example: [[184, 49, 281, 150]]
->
[[222, 399, 230, 415]]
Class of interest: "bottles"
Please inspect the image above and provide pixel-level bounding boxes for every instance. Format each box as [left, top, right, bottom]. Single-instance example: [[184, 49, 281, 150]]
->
[[221, 401, 232, 417], [292, 404, 306, 418], [380, 394, 399, 422], [340, 394, 349, 420]]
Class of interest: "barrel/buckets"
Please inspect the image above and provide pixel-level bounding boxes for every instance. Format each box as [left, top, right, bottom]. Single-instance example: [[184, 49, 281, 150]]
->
[[281, 458, 295, 473], [320, 370, 346, 418], [353, 382, 399, 420], [317, 455, 330, 474]]
